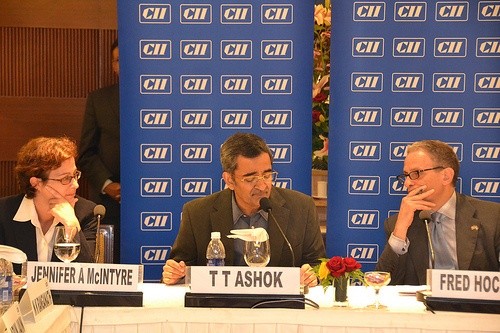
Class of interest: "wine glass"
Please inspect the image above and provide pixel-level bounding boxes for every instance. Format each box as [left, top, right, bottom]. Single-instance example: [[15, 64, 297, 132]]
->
[[54, 225, 81, 264], [364, 271, 391, 311]]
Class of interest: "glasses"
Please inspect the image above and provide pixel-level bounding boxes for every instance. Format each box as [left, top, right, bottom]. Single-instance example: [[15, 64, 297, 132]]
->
[[45, 170, 82, 185], [396, 166, 445, 183], [231, 170, 278, 185]]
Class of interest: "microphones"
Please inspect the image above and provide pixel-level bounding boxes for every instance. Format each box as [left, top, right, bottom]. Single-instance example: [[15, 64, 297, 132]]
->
[[93, 204, 106, 262], [259, 197, 295, 266], [419, 209, 435, 269]]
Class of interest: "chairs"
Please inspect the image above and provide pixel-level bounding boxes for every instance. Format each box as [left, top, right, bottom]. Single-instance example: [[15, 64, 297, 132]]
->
[[98, 224, 114, 263]]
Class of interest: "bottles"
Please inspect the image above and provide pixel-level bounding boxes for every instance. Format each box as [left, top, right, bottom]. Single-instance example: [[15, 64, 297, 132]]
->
[[205, 232, 226, 267], [0, 257, 14, 314]]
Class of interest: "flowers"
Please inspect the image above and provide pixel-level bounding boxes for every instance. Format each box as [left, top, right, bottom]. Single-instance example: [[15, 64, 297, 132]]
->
[[309, 256, 368, 302], [312, 4, 332, 172]]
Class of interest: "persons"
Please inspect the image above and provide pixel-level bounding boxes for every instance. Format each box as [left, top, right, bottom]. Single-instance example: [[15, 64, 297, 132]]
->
[[375, 139, 500, 285], [0, 137, 98, 276], [161, 132, 329, 287], [76, 39, 121, 263]]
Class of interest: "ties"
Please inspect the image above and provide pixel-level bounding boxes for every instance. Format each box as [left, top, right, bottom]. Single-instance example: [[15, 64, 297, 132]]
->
[[431, 213, 454, 269]]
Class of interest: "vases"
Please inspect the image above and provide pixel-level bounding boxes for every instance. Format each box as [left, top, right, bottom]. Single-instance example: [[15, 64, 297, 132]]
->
[[313, 169, 327, 199], [334, 278, 348, 307]]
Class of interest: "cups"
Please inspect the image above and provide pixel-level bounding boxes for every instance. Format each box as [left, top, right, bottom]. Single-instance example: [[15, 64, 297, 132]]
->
[[98, 225, 115, 264], [244, 240, 271, 268]]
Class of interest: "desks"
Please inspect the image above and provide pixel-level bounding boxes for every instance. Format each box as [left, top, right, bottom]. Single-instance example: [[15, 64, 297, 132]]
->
[[21, 283, 500, 333]]
